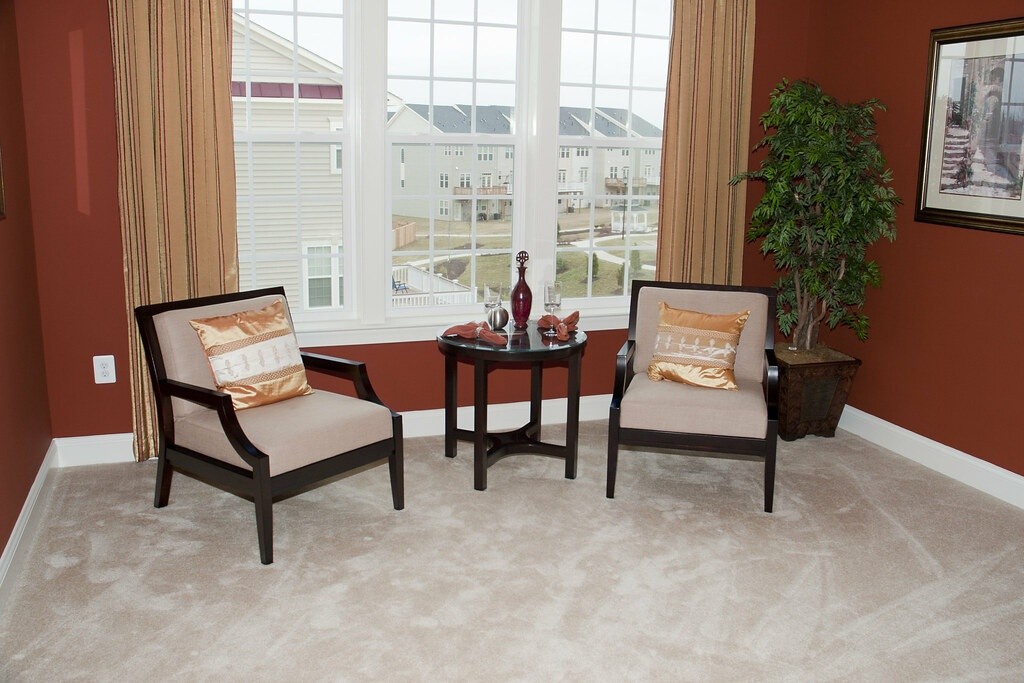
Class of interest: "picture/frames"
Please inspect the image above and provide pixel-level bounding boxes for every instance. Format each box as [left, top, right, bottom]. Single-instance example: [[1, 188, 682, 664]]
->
[[914, 16, 1024, 239]]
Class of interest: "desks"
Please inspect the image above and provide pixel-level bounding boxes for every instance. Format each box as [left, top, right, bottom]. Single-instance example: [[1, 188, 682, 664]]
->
[[437, 318, 589, 491]]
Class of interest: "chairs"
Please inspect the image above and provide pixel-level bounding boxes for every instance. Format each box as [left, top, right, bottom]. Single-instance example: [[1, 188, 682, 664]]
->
[[132, 287, 405, 564], [606, 280, 780, 514]]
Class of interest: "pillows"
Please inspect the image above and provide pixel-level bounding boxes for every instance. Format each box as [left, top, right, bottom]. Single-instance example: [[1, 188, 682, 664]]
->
[[190, 296, 317, 412], [647, 301, 751, 393]]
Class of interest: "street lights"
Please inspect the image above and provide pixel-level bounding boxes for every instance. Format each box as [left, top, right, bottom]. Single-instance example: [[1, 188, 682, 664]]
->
[[621, 176, 628, 240]]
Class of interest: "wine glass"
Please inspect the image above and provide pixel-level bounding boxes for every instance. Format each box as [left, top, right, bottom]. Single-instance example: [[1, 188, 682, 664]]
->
[[543, 281, 560, 336], [483, 282, 502, 331]]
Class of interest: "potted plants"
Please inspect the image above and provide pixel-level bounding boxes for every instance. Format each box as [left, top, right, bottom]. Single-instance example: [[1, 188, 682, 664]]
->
[[727, 71, 905, 442]]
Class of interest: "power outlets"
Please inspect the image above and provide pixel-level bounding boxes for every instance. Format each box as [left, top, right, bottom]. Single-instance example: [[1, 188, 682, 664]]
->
[[93, 355, 117, 384]]
